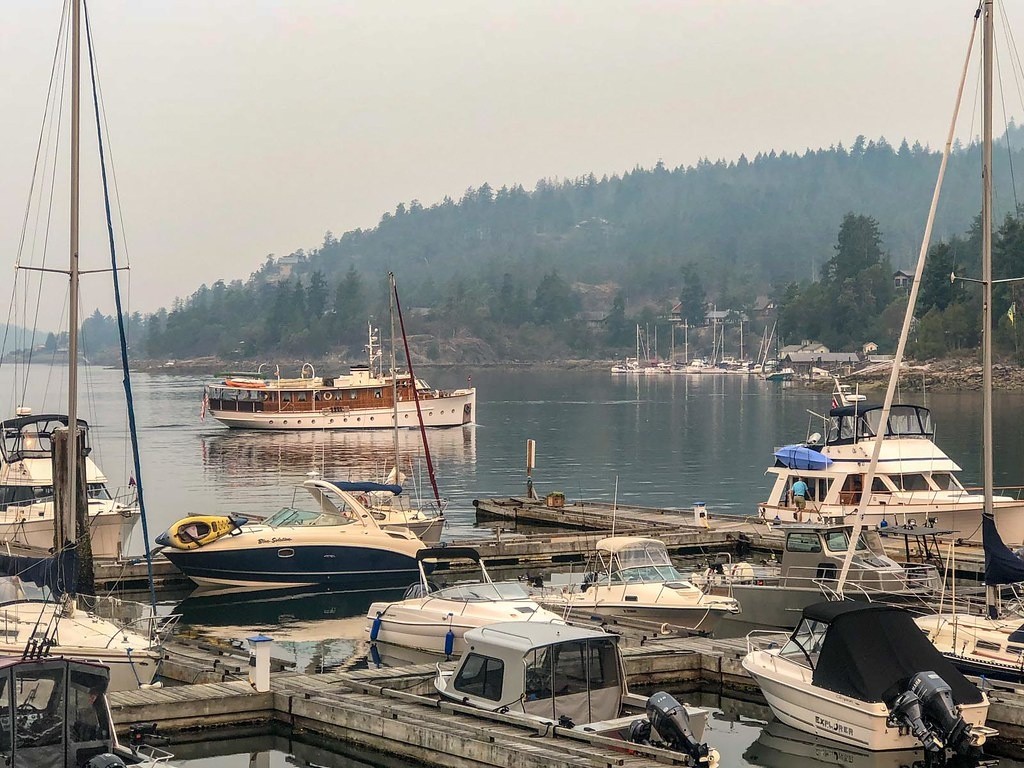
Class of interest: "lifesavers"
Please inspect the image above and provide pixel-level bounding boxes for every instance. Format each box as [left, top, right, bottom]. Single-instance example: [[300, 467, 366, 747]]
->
[[358, 496, 368, 507], [323, 391, 332, 400], [465, 405, 470, 412]]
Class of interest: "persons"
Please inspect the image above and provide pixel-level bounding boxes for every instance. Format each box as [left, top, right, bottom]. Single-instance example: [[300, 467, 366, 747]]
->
[[263, 393, 269, 401], [790, 476, 813, 522]]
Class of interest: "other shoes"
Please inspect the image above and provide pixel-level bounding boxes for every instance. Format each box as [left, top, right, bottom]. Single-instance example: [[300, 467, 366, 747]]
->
[[794, 512, 797, 519]]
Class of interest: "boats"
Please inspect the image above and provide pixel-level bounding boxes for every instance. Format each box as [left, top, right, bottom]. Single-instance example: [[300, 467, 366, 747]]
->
[[201, 269, 478, 430], [157, 584, 407, 642], [769, 521, 945, 594], [335, 472, 447, 541], [439, 622, 709, 761], [0, 576, 167, 694], [739, 599, 992, 751], [364, 546, 570, 660], [154, 478, 439, 586], [522, 535, 743, 636], [0, 658, 177, 768], [910, 611, 1024, 684], [754, 372, 1024, 549]]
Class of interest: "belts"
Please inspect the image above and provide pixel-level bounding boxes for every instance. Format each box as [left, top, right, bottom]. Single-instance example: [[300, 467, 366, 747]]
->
[[794, 495, 803, 497]]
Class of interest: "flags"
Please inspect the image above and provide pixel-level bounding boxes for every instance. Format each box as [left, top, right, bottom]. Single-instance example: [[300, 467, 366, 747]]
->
[[831, 398, 841, 422], [200, 388, 209, 422]]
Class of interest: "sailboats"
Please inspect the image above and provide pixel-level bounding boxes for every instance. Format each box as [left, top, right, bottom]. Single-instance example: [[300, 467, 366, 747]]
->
[[609, 314, 794, 382], [761, 2, 1024, 678], [0, 0, 143, 570]]
